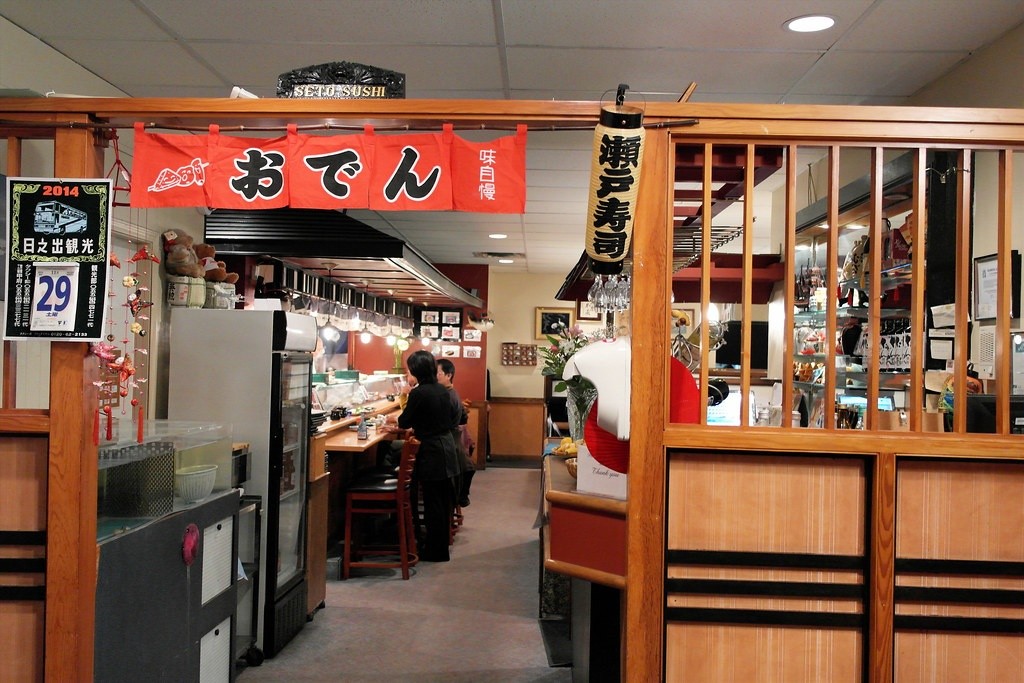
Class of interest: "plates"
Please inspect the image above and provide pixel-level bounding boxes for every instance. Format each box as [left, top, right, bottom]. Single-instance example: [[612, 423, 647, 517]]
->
[[310, 410, 327, 435]]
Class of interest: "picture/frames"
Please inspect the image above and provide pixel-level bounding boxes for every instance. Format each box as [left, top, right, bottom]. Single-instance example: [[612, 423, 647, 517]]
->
[[575, 299, 602, 322], [534, 306, 577, 341]]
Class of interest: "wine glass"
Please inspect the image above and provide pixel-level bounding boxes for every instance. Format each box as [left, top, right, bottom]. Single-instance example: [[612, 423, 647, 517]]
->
[[587, 270, 634, 312]]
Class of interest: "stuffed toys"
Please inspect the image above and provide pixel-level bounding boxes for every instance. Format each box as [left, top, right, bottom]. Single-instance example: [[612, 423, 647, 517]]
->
[[191, 243, 239, 283], [164, 229, 206, 278]]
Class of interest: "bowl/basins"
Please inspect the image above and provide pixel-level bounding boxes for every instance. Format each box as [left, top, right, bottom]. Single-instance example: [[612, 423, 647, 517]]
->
[[176, 464, 218, 502]]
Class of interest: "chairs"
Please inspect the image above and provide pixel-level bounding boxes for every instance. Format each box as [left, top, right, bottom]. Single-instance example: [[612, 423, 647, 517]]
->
[[342, 437, 421, 580]]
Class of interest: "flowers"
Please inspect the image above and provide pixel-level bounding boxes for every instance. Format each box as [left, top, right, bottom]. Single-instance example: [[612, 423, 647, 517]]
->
[[534, 317, 619, 435]]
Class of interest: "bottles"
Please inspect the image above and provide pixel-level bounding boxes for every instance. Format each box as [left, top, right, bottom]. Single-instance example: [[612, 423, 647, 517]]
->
[[860, 316, 918, 372], [356, 412, 368, 440], [836, 403, 859, 432], [280, 454, 297, 493]]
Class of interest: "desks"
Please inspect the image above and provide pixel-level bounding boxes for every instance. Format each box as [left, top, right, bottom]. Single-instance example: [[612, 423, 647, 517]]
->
[[323, 407, 405, 452]]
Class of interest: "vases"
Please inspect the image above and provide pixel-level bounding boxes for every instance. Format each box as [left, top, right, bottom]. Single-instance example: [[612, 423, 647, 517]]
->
[[565, 387, 599, 444]]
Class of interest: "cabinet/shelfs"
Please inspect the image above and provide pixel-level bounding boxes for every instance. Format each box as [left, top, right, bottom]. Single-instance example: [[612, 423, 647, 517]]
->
[[236, 494, 263, 669], [792, 263, 912, 399]]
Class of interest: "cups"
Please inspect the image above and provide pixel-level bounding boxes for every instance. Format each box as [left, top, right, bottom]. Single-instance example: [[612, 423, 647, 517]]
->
[[331, 406, 347, 420]]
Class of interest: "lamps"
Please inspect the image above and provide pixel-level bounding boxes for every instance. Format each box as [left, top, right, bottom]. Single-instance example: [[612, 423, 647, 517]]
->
[[322, 263, 430, 352]]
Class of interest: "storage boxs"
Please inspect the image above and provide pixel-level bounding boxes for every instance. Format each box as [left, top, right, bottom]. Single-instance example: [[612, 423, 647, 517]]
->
[[575, 444, 628, 500]]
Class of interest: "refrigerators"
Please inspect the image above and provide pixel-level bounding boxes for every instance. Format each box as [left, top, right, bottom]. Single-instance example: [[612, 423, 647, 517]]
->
[[167, 309, 320, 662]]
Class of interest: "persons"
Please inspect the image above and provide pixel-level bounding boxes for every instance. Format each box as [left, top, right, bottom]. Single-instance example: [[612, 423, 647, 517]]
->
[[378, 350, 475, 563]]
